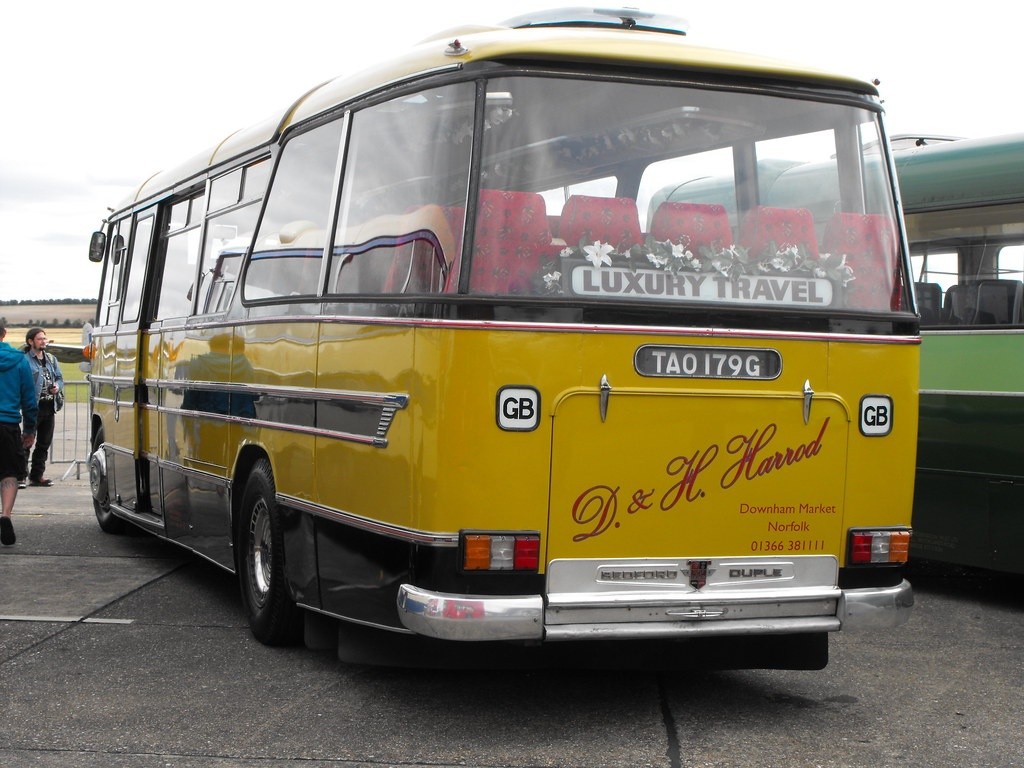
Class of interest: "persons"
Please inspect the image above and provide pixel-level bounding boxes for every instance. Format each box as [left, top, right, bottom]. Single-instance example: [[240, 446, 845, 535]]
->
[[15, 327, 64, 489], [169, 332, 259, 481], [0, 317, 40, 546]]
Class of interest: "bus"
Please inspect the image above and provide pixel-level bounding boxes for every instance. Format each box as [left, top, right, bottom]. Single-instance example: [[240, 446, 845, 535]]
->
[[79, 4, 925, 669], [644, 131, 1024, 611]]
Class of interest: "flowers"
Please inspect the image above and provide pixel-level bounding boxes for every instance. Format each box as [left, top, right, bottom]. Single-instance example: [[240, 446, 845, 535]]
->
[[614, 247, 630, 261], [642, 233, 701, 276], [757, 241, 803, 278], [560, 233, 613, 268], [708, 245, 750, 277], [534, 258, 560, 293], [817, 253, 855, 289]]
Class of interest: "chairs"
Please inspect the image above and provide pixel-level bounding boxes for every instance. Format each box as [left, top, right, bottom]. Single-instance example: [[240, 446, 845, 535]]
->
[[737, 207, 817, 260], [650, 202, 733, 257], [447, 191, 552, 291], [915, 277, 1023, 328], [824, 214, 901, 312], [385, 205, 462, 297], [557, 195, 644, 245]]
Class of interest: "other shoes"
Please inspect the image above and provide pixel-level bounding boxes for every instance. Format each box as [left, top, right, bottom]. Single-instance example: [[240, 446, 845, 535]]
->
[[30, 478, 54, 487], [1, 515, 15, 544], [17, 480, 26, 488]]
